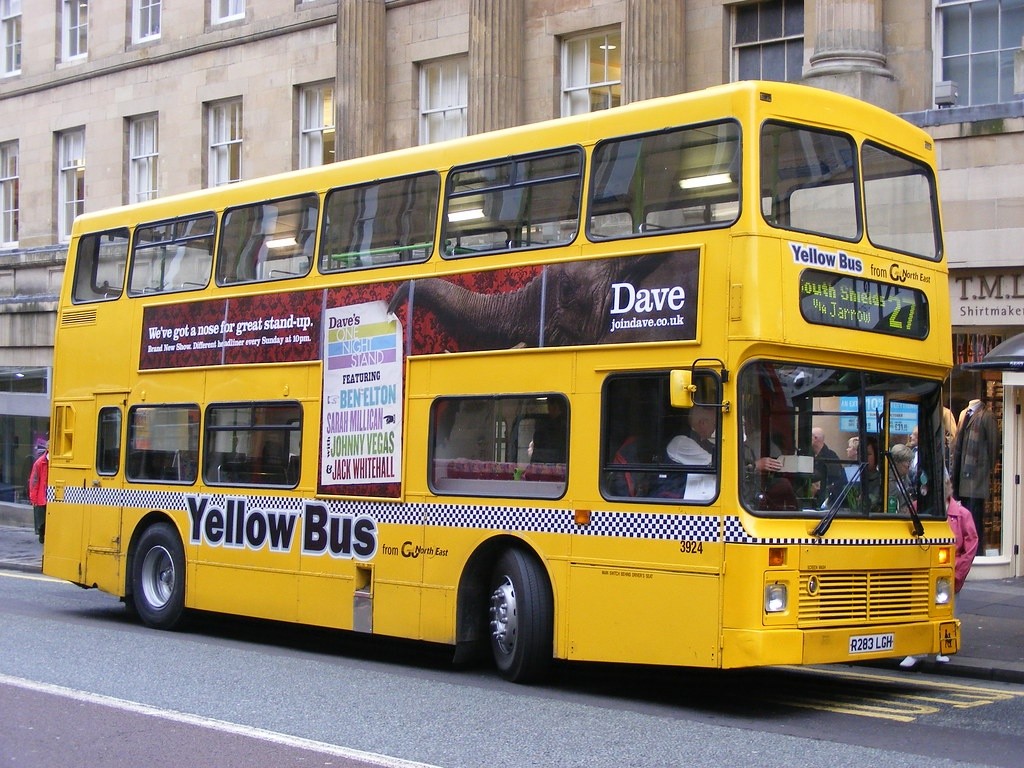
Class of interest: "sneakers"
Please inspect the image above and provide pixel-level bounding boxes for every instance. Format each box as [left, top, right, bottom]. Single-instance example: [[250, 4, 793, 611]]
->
[[899, 656, 922, 666], [936, 654, 949, 662]]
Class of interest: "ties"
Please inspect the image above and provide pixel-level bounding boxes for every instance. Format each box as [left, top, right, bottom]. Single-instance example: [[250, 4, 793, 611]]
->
[[959, 409, 973, 444]]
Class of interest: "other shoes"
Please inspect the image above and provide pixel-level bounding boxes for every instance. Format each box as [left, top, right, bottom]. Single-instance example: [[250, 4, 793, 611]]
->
[[39, 535, 44, 544]]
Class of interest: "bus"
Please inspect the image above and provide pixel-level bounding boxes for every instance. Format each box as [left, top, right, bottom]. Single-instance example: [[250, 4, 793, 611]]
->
[[42, 79, 963, 684]]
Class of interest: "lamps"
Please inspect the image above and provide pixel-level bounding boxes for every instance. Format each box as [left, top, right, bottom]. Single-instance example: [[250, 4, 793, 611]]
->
[[677, 172, 733, 189]]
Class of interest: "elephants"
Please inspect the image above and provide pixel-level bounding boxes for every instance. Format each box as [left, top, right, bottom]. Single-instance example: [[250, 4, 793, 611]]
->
[[387, 246, 700, 351]]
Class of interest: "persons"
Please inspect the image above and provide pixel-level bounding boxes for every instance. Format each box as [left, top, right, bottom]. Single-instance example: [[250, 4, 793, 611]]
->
[[743, 417, 798, 510], [897, 464, 980, 666], [28, 439, 50, 544], [809, 426, 918, 513], [952, 399, 999, 554], [666, 401, 719, 501], [942, 405, 956, 450], [528, 394, 570, 464]]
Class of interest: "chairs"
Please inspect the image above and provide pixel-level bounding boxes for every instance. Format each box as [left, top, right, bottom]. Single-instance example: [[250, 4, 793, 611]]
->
[[104, 270, 294, 299], [105, 449, 299, 486]]
